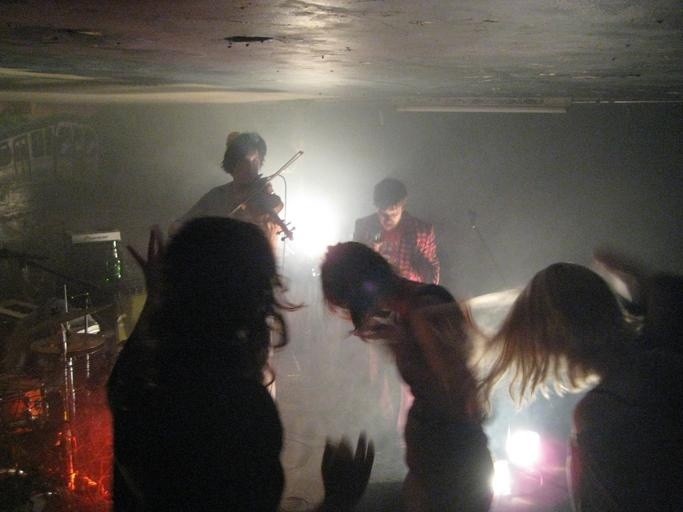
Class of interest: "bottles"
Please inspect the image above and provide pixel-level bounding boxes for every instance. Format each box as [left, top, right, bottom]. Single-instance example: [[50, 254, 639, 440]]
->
[[104, 240, 122, 283]]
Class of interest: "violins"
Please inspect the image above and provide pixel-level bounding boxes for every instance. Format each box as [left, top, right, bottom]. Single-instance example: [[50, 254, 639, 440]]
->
[[237, 179, 294, 240]]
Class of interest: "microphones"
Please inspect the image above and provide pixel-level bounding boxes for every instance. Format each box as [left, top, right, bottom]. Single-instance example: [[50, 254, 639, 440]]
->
[[1, 249, 45, 260]]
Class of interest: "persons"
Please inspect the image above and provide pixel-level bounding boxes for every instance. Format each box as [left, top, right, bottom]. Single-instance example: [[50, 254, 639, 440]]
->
[[478, 244, 683, 512], [351, 173, 440, 473], [167, 128, 284, 409], [102, 213, 376, 512], [319, 240, 497, 512]]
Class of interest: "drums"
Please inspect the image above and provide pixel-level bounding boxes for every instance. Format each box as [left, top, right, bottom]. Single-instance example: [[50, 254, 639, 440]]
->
[[0, 373, 48, 433]]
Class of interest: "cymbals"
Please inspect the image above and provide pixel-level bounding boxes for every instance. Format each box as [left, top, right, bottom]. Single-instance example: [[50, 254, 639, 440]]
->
[[22, 303, 112, 337], [31, 333, 106, 360]]
